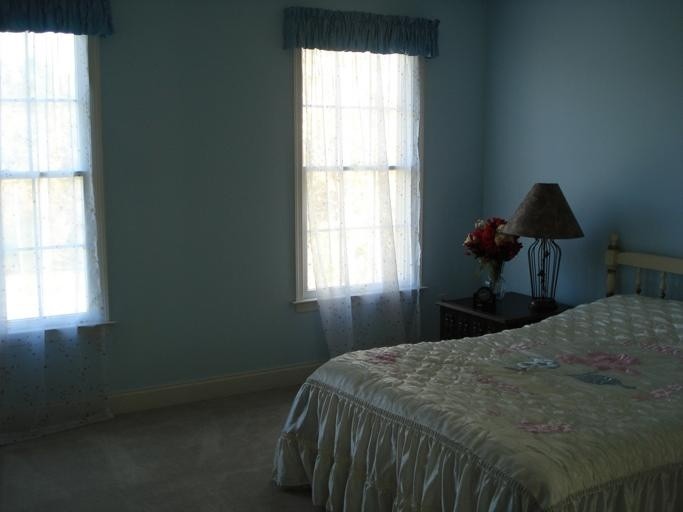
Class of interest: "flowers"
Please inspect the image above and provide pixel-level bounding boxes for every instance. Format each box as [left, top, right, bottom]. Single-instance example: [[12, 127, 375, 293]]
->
[[461, 217, 523, 276]]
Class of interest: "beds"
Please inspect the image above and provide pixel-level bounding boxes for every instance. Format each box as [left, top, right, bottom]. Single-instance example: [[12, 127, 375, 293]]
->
[[272, 232, 683, 512]]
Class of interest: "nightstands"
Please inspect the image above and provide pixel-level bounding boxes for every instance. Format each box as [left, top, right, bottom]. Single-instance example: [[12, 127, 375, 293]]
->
[[433, 291, 575, 341]]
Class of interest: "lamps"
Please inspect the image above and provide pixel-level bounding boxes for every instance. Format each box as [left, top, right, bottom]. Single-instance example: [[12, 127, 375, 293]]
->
[[499, 183, 584, 313]]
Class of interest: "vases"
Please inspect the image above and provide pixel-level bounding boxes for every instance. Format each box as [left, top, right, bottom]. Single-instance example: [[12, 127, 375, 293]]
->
[[484, 275, 504, 300]]
